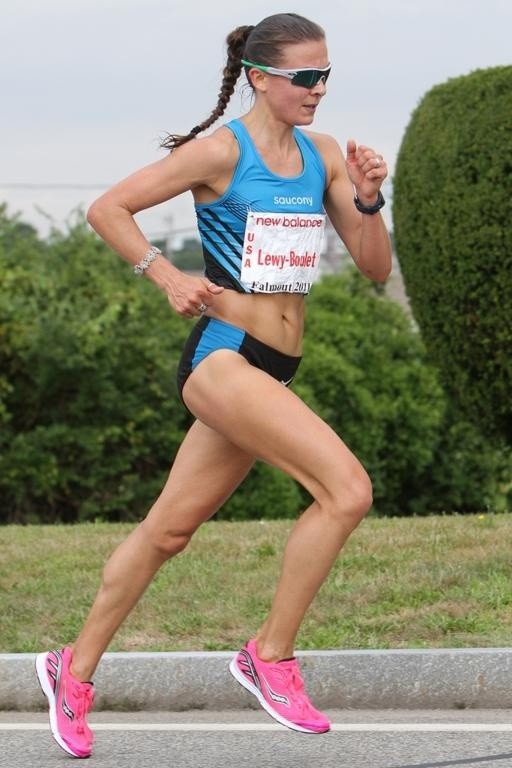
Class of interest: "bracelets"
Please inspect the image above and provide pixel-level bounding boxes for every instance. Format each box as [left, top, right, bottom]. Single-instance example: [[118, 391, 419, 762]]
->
[[133, 245, 162, 276], [353, 189, 386, 214]]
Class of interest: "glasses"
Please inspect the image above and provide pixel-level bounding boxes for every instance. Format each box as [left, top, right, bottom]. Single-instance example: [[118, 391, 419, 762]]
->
[[240, 59, 333, 89]]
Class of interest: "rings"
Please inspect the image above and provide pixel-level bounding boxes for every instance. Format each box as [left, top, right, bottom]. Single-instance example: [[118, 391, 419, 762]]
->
[[375, 158, 380, 169], [207, 282, 215, 288], [198, 302, 209, 313]]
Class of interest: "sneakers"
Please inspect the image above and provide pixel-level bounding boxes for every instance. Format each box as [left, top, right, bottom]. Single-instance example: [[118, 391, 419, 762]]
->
[[36, 646, 96, 758], [227, 638, 331, 734]]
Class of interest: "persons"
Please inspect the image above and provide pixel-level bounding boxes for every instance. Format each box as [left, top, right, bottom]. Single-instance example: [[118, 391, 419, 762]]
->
[[29, 12, 395, 757]]
